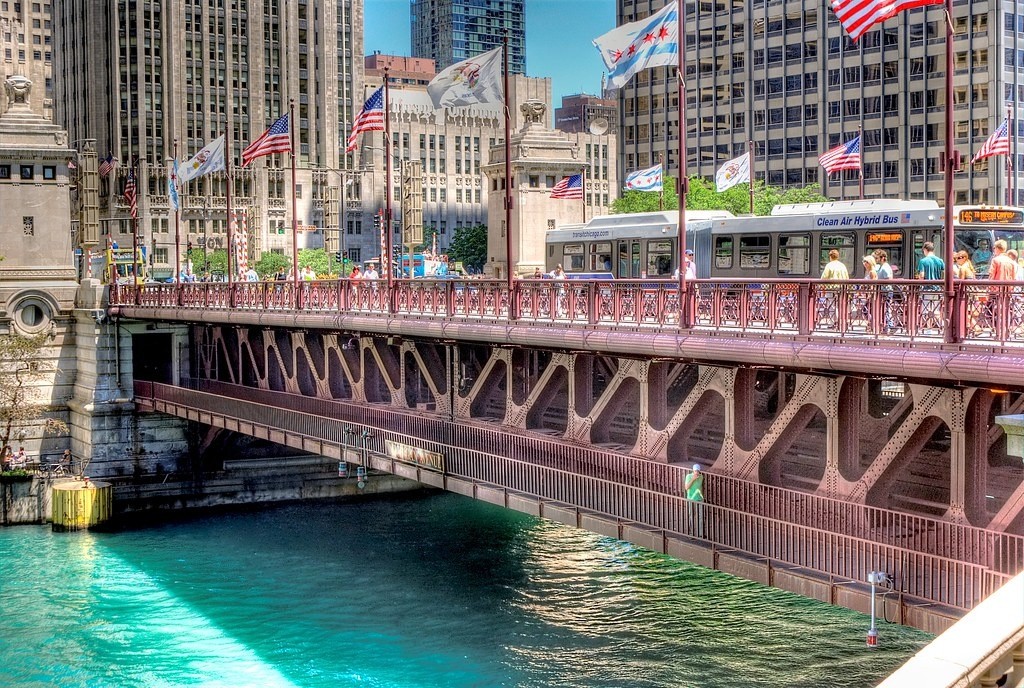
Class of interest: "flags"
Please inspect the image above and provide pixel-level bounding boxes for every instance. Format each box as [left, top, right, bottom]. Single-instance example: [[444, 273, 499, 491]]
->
[[624, 163, 663, 194], [969, 117, 1009, 166], [241, 112, 292, 167], [168, 159, 179, 211], [176, 134, 225, 184], [716, 152, 751, 192], [818, 136, 860, 176], [124, 166, 135, 219], [346, 85, 385, 153], [427, 46, 504, 110], [830, 0, 945, 45], [592, 0, 680, 88], [98, 154, 115, 176], [549, 174, 583, 200]]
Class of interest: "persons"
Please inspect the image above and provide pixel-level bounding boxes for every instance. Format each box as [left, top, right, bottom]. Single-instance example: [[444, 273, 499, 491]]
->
[[819, 237, 1024, 341], [349, 241, 703, 325], [58, 448, 72, 473], [111, 241, 317, 307], [0, 445, 29, 471], [685, 463, 708, 540]]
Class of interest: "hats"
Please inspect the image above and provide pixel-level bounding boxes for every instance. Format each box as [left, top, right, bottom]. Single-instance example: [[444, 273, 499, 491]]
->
[[693, 463, 700, 471], [684, 257, 690, 262], [863, 255, 876, 267], [684, 249, 694, 254]]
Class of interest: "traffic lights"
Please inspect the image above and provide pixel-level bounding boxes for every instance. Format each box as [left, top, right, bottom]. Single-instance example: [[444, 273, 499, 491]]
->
[[373, 215, 379, 228], [187, 242, 194, 254], [335, 251, 342, 263], [154, 238, 156, 248], [207, 261, 211, 271], [343, 251, 348, 264], [279, 223, 284, 235]]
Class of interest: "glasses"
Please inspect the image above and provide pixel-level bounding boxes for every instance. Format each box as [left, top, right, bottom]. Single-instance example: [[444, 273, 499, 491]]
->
[[957, 256, 964, 259], [688, 254, 693, 256]]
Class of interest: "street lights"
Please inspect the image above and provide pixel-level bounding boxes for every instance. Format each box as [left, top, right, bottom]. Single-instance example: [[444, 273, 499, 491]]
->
[[132, 156, 147, 300], [364, 145, 404, 278], [307, 161, 345, 275]]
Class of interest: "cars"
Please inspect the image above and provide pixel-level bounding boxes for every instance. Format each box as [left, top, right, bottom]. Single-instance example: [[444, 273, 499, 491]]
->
[[411, 274, 504, 301]]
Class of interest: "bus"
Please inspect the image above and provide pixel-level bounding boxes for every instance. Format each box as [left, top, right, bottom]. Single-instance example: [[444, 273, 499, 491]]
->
[[397, 252, 449, 279], [544, 198, 1023, 329], [91, 248, 147, 293]]
